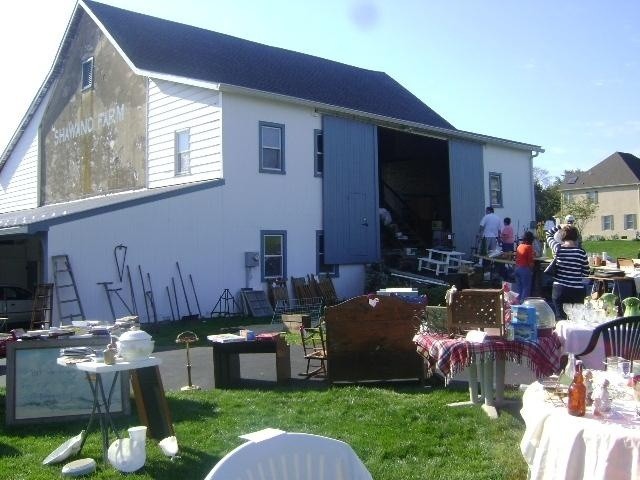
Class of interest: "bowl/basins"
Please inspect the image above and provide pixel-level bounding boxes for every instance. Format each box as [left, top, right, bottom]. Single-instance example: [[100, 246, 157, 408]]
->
[[26, 325, 75, 340]]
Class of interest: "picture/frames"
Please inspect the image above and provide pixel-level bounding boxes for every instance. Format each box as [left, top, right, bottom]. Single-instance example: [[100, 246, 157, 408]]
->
[[3, 337, 135, 428]]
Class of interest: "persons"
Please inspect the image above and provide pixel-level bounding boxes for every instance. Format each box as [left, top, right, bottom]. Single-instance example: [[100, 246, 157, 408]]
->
[[500, 217, 514, 261], [514, 231, 536, 303], [545, 223, 590, 320], [479, 207, 501, 251], [553, 215, 579, 245]]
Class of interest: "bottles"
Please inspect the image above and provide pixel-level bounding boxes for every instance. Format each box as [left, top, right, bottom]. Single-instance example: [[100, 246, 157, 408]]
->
[[560, 351, 640, 418]]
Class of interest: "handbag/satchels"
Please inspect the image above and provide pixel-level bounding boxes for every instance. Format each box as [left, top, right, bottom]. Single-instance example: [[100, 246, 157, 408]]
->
[[544, 244, 560, 275]]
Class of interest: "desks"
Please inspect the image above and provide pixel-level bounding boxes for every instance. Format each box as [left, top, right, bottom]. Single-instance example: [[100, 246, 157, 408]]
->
[[411, 326, 558, 421], [416, 243, 640, 299], [517, 368, 640, 480], [212, 331, 292, 389], [555, 316, 640, 366], [55, 353, 164, 466]]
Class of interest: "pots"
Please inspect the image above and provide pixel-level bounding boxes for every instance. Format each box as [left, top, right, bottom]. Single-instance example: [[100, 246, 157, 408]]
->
[[116, 326, 157, 361]]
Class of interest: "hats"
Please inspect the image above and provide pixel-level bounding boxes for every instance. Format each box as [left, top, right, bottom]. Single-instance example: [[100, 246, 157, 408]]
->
[[565, 214, 575, 221]]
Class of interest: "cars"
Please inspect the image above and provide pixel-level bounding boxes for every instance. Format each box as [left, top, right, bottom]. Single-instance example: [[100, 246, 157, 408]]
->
[[0, 284, 41, 327]]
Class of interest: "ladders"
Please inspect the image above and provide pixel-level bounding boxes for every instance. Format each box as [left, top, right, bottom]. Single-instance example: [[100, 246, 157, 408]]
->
[[52, 254, 86, 324], [30, 283, 53, 330]]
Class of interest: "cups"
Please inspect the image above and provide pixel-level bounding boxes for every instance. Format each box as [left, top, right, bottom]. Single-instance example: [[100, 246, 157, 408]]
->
[[586, 251, 609, 268], [96, 351, 105, 362]]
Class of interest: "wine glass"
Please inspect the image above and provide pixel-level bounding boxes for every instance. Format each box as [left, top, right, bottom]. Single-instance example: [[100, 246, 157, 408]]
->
[[561, 298, 607, 324]]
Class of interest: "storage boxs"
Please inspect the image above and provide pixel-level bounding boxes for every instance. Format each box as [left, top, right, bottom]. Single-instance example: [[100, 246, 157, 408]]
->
[[509, 304, 542, 327], [505, 321, 541, 344]]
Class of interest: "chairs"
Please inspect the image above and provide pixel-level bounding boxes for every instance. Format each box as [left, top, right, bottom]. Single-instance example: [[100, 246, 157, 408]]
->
[[203, 431, 375, 479], [572, 315, 640, 363], [299, 326, 330, 379]]
